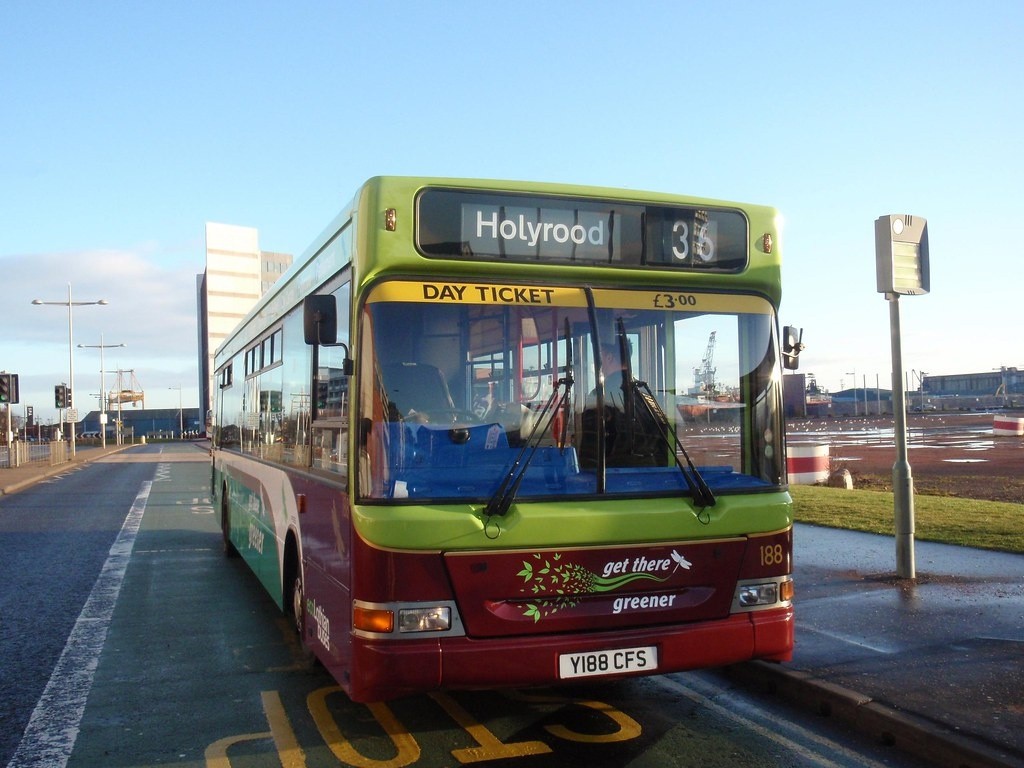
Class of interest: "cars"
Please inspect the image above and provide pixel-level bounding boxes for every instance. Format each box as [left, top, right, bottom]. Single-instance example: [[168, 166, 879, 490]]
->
[[913, 404, 937, 411]]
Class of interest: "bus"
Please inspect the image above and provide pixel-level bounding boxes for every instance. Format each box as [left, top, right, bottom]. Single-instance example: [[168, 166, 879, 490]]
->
[[206, 173, 808, 700]]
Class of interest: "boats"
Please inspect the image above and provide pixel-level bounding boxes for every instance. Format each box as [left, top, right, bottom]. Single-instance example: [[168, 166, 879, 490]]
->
[[675, 371, 836, 424]]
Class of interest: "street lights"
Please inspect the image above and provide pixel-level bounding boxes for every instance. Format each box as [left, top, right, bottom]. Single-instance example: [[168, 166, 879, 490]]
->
[[100, 370, 135, 446], [30, 299, 109, 456], [90, 391, 108, 437], [169, 387, 183, 439], [78, 342, 128, 449]]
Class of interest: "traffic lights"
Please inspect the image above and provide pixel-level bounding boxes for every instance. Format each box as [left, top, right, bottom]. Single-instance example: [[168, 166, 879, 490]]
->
[[317, 380, 328, 409], [55, 385, 66, 409], [0, 370, 12, 404], [66, 388, 71, 408]]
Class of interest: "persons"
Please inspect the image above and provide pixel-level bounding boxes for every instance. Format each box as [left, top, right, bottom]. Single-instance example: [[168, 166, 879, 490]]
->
[[580, 334, 668, 470]]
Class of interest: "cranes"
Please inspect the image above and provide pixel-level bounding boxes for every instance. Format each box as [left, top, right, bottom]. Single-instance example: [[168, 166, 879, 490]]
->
[[692, 331, 716, 392]]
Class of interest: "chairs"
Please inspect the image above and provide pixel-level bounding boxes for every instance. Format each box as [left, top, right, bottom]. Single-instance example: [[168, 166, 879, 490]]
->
[[381, 361, 456, 425]]
